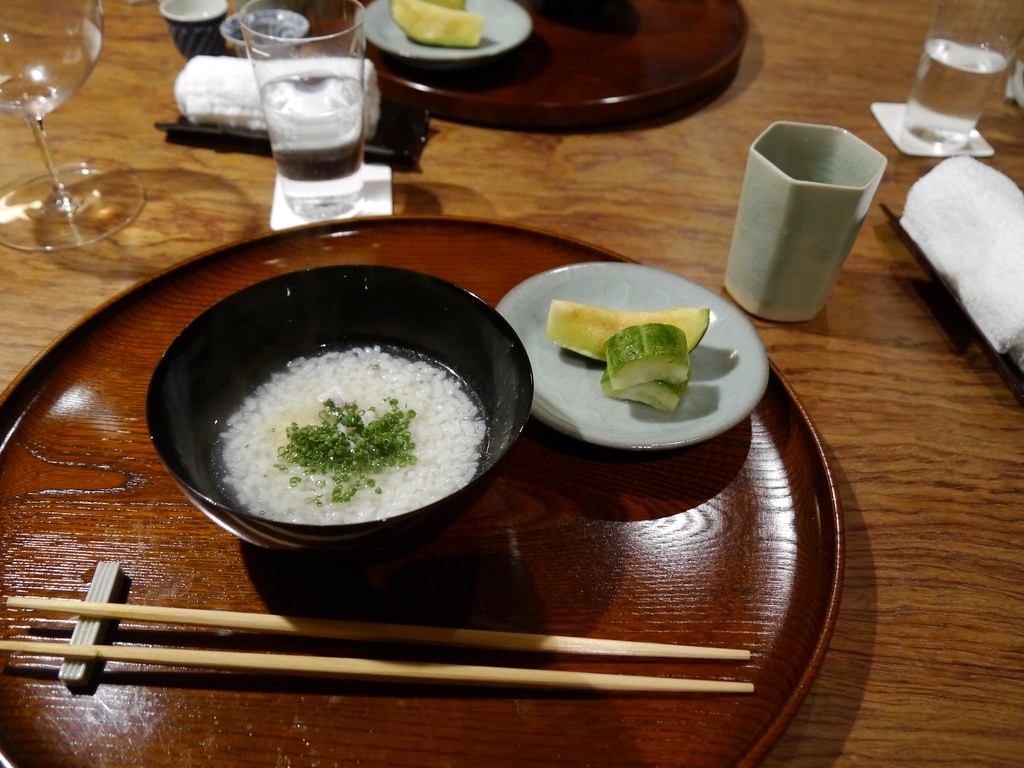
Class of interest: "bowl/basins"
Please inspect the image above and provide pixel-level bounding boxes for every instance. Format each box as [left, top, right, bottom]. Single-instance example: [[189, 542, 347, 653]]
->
[[146, 265, 535, 575]]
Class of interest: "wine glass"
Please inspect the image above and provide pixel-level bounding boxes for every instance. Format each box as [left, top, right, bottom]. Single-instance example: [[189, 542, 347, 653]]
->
[[0, 0, 146, 251]]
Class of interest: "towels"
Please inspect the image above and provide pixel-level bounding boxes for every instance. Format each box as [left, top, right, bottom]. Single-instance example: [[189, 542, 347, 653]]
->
[[175, 55, 380, 141], [901, 156, 1024, 368]]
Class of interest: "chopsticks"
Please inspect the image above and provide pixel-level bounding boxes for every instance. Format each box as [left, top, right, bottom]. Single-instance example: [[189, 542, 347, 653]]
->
[[0, 594, 758, 697]]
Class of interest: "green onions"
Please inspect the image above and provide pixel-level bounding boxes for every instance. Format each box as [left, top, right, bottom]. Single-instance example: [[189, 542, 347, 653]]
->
[[272, 398, 418, 508]]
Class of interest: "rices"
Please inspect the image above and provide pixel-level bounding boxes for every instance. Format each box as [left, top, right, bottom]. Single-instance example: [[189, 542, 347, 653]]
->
[[217, 346, 486, 526]]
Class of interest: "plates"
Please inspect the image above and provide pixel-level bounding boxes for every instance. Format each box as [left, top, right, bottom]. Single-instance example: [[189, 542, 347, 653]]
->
[[354, 0, 533, 73], [495, 261, 770, 451]]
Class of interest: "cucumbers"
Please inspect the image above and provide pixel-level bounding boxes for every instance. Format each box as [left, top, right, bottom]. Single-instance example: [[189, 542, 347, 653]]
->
[[601, 366, 692, 412], [605, 322, 691, 391]]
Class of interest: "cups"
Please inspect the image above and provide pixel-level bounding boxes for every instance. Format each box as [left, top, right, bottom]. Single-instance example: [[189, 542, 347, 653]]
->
[[240, 0, 368, 221], [220, 9, 311, 61], [159, 0, 230, 62], [723, 120, 888, 323], [902, 0, 1024, 148]]
